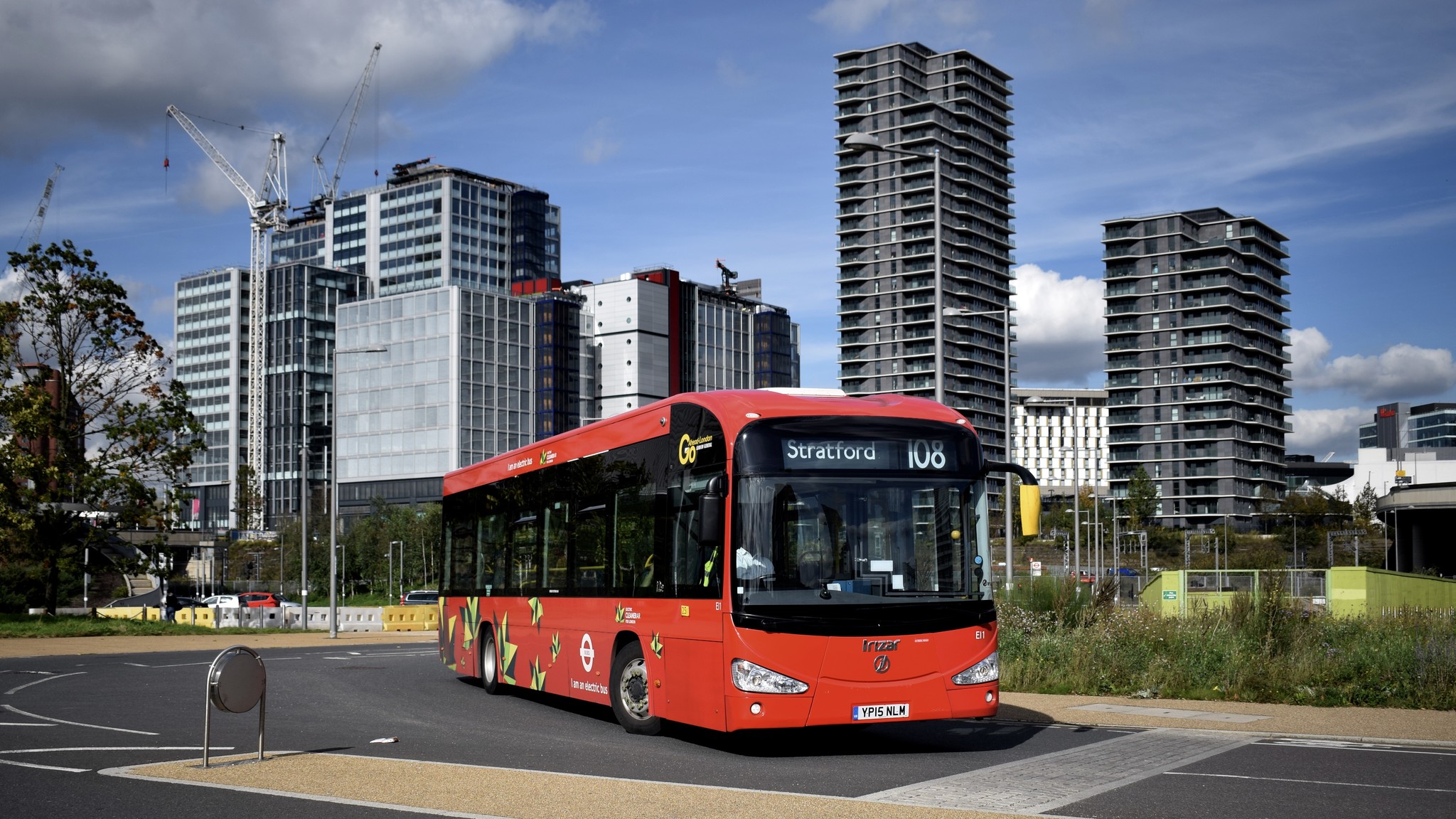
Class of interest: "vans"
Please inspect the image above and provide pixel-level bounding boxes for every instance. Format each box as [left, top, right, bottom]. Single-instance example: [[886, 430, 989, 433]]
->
[[1107, 568, 1141, 576]]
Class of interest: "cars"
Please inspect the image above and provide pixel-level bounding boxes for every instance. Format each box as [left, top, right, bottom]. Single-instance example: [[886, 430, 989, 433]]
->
[[173, 592, 302, 609]]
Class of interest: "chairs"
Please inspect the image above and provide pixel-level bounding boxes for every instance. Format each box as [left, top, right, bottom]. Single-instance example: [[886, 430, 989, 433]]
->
[[451, 550, 831, 597]]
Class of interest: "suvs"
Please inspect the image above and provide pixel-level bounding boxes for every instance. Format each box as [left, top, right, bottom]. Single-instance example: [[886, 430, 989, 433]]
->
[[1070, 570, 1096, 582], [401, 590, 439, 605]]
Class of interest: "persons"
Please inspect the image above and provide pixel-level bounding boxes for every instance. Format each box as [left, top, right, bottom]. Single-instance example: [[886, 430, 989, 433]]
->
[[163, 589, 178, 624], [1183, 377, 1187, 382], [453, 543, 653, 589], [736, 525, 776, 581], [820, 542, 852, 580], [1187, 377, 1192, 382]]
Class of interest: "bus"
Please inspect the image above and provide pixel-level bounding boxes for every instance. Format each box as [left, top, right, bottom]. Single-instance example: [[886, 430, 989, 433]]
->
[[439, 387, 1040, 737]]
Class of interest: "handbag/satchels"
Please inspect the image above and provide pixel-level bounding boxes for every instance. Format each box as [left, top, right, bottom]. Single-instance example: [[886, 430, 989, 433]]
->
[[175, 602, 183, 611]]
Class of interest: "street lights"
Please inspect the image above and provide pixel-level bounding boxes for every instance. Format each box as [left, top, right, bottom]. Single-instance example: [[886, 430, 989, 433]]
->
[[940, 306, 1014, 598], [1088, 492, 1117, 599], [336, 545, 344, 606], [1024, 397, 1082, 595], [330, 348, 387, 639], [1395, 412, 1410, 486], [843, 132, 943, 406], [1081, 521, 1104, 591], [1064, 509, 1091, 579], [1224, 516, 1229, 577], [1058, 446, 1099, 589], [1288, 515, 1297, 596], [389, 540, 404, 607]]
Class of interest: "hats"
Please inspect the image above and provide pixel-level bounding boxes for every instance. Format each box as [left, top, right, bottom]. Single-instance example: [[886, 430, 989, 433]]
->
[[167, 588, 173, 593]]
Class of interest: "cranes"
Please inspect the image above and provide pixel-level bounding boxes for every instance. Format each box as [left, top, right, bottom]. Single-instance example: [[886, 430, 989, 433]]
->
[[309, 41, 383, 211], [163, 103, 292, 228]]
